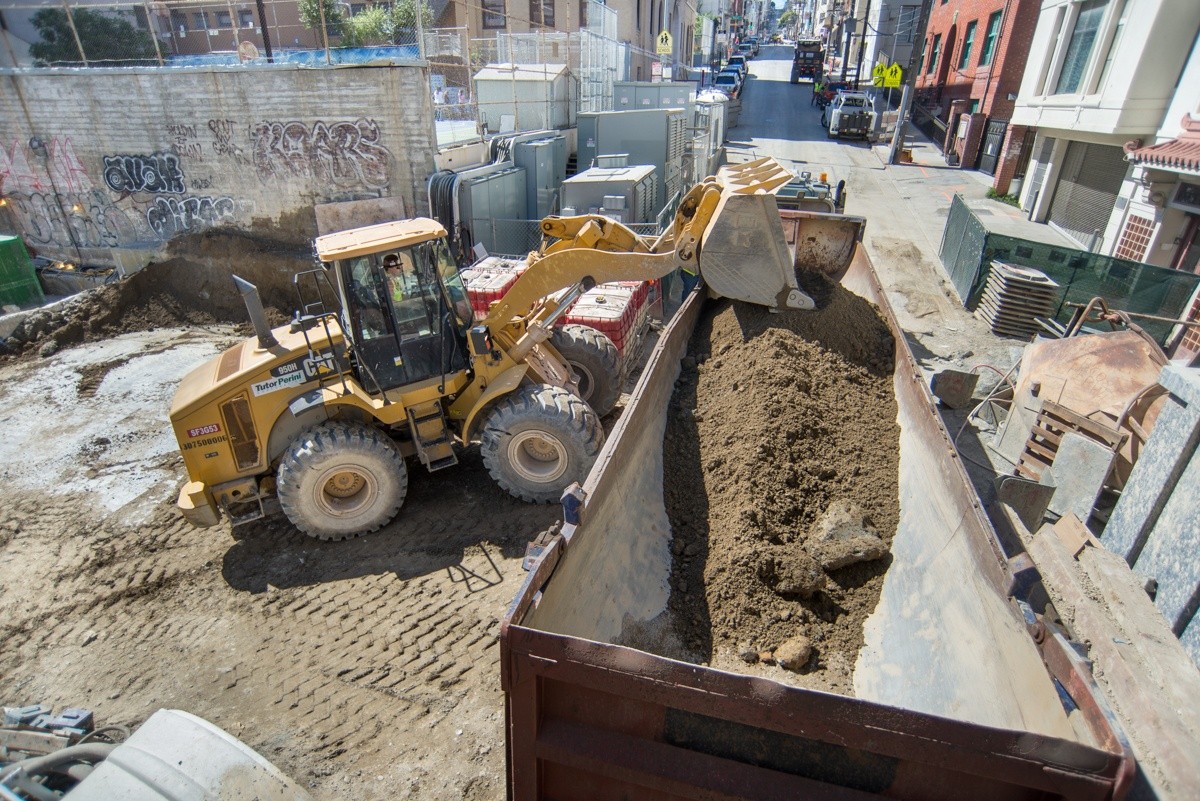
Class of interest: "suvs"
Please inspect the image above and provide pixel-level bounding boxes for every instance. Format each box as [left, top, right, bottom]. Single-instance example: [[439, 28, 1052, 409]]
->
[[821, 90, 878, 139]]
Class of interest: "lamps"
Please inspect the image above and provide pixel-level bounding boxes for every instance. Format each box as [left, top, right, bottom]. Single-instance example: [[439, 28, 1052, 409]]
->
[[1008, 93, 1017, 101]]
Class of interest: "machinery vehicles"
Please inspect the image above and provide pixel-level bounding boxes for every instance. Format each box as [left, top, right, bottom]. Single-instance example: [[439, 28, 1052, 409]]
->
[[166, 155, 846, 543]]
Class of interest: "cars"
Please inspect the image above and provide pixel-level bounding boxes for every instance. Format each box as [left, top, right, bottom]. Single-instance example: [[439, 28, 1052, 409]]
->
[[715, 55, 749, 99], [736, 36, 761, 58], [816, 82, 852, 109]]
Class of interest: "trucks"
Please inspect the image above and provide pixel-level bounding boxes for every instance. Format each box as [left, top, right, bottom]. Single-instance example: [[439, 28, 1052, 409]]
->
[[790, 38, 826, 85], [499, 168, 1135, 801]]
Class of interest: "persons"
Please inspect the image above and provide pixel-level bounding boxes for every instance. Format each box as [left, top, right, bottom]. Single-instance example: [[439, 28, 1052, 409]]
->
[[773, 33, 777, 47], [381, 255, 433, 316], [811, 77, 819, 107]]
